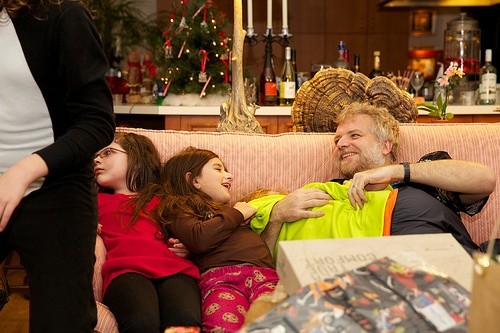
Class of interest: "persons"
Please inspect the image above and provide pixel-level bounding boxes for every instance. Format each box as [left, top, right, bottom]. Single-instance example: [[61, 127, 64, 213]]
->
[[93, 105, 500, 333], [0, 0, 116, 333]]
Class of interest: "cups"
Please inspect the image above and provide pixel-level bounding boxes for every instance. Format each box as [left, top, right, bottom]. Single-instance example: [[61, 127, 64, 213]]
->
[[311, 63, 325, 79]]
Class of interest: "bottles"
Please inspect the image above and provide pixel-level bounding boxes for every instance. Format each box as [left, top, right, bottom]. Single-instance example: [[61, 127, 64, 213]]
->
[[261, 45, 277, 106], [368, 51, 383, 79], [335, 40, 351, 70], [291, 49, 299, 90], [352, 54, 360, 72], [279, 47, 296, 107], [344, 49, 350, 63], [478, 49, 497, 105], [442, 13, 482, 106]]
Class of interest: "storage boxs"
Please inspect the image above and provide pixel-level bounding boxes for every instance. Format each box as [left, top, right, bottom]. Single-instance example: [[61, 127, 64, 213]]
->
[[276, 233, 475, 297]]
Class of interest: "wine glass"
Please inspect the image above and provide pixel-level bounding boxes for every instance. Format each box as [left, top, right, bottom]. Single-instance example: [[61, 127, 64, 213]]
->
[[410, 71, 425, 97]]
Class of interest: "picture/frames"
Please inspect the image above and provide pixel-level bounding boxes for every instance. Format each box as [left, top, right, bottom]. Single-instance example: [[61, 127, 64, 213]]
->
[[408, 12, 437, 36]]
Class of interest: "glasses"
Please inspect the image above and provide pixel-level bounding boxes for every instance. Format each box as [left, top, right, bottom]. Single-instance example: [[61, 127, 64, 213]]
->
[[94, 147, 127, 158]]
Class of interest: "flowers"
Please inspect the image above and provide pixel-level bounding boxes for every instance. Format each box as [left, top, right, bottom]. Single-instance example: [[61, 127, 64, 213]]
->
[[417, 62, 465, 119]]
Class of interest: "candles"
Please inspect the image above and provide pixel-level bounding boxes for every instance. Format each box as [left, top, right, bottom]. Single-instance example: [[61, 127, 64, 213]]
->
[[282, 0, 288, 28], [246, 0, 253, 29], [266, 0, 273, 28]]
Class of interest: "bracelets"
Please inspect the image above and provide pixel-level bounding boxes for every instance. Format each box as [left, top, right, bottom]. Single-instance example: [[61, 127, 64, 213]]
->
[[400, 162, 411, 184]]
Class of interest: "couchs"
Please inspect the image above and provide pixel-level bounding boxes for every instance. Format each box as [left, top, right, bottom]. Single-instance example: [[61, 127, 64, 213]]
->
[[92, 123, 500, 333]]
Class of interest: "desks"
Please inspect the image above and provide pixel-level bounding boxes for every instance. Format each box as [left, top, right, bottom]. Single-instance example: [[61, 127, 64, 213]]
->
[[114, 100, 500, 130]]
